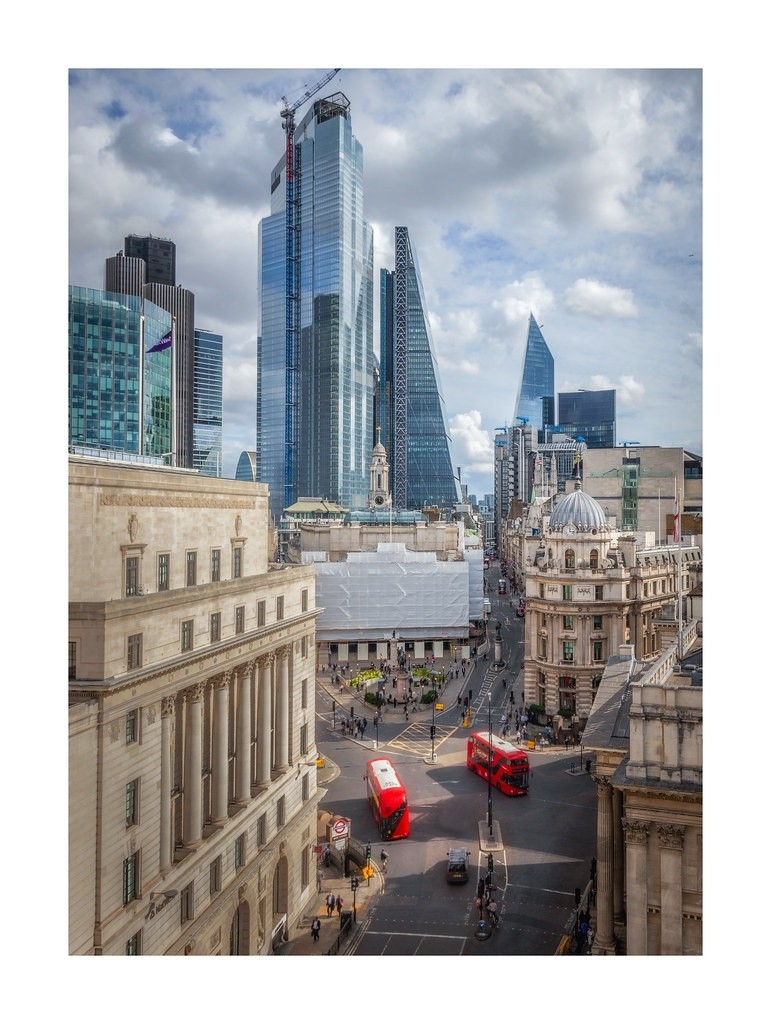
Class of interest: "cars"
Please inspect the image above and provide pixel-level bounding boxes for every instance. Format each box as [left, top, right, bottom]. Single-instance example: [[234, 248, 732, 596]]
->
[[484, 542, 498, 571]]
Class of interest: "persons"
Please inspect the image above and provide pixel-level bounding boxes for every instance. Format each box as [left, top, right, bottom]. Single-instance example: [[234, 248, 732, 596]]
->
[[501, 709, 583, 751], [341, 717, 367, 739], [502, 678, 505, 688], [310, 917, 321, 944], [481, 546, 526, 607], [325, 848, 331, 868], [586, 758, 592, 771], [373, 716, 378, 725], [316, 646, 487, 721], [325, 892, 343, 917], [484, 887, 498, 920], [457, 696, 462, 708], [380, 849, 389, 874], [316, 868, 323, 892], [574, 905, 595, 952]]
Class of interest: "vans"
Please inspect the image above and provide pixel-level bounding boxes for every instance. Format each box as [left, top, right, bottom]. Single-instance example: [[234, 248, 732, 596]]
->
[[446, 846, 471, 886]]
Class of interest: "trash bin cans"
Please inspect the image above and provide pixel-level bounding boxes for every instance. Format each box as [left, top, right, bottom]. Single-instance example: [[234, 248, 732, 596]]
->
[[340, 910, 351, 930]]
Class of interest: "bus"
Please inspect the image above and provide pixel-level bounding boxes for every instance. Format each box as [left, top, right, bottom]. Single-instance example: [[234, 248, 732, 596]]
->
[[467, 730, 534, 798], [363, 756, 410, 841], [498, 578, 507, 595]]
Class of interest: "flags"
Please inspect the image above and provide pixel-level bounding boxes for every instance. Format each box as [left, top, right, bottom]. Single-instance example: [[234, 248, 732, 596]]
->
[[145, 330, 172, 353]]
